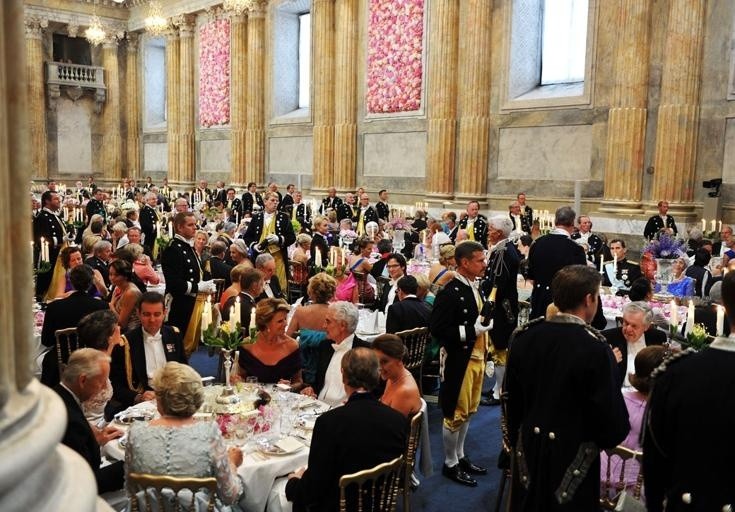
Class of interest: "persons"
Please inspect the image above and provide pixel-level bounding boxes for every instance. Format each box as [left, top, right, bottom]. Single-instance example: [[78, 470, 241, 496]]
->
[[31, 171, 735, 511]]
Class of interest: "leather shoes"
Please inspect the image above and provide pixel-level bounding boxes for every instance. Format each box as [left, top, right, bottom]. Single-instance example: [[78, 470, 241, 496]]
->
[[459, 455, 486, 474], [442, 462, 476, 486], [479, 389, 500, 406]]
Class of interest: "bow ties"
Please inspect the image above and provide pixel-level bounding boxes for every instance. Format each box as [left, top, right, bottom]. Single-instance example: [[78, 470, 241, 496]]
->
[[331, 343, 345, 350], [265, 213, 273, 218], [469, 281, 479, 289], [389, 281, 396, 287], [466, 219, 474, 223], [146, 334, 161, 342]]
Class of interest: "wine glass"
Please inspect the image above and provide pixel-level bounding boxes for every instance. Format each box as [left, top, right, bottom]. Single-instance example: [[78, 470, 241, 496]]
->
[[155, 264, 162, 273], [598, 286, 631, 311], [354, 303, 366, 316], [143, 376, 292, 458]]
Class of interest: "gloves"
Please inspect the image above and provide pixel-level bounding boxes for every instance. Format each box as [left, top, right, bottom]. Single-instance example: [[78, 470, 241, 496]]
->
[[63, 232, 75, 243], [475, 316, 493, 336], [254, 243, 265, 253], [159, 215, 168, 228], [198, 280, 216, 294], [485, 361, 494, 378], [266, 235, 279, 246]]
